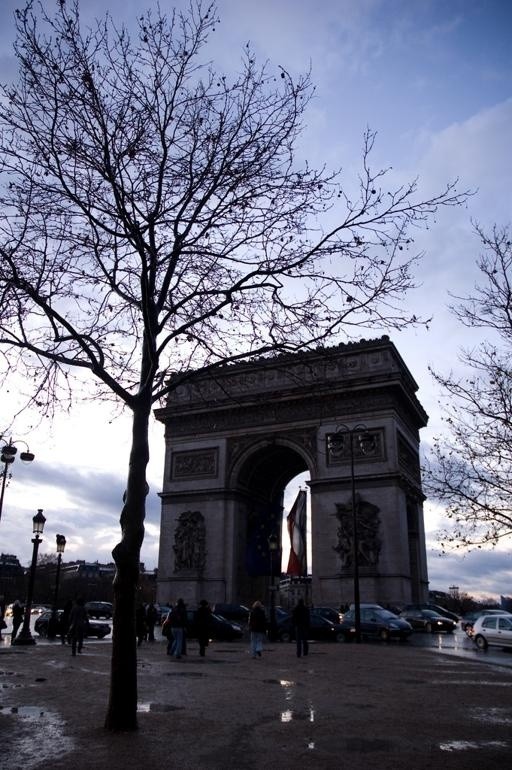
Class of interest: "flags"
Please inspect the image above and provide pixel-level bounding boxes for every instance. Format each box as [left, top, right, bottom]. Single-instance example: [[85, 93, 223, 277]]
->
[[284, 489, 309, 578]]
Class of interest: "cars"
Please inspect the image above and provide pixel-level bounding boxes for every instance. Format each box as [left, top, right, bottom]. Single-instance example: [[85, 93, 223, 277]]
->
[[399, 609, 457, 634], [461, 609, 511, 637], [162, 609, 244, 643], [153, 606, 171, 625], [265, 606, 288, 622], [340, 608, 414, 641], [23, 606, 26, 615], [35, 610, 111, 639], [268, 615, 356, 643], [30, 606, 46, 614], [213, 603, 252, 623]]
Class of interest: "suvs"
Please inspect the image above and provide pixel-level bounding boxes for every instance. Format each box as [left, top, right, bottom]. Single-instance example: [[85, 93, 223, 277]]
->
[[402, 602, 459, 624], [470, 614, 512, 650], [308, 608, 344, 624], [85, 601, 113, 619]]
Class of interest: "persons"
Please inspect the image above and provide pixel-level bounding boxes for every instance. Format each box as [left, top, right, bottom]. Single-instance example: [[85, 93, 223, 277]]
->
[[137, 597, 188, 659], [11, 600, 25, 642], [68, 597, 91, 657], [0, 593, 8, 641], [194, 599, 213, 657], [291, 597, 311, 658], [248, 600, 267, 659]]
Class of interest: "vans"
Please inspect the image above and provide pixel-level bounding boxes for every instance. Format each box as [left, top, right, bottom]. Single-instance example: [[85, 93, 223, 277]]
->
[[348, 604, 382, 611], [6, 603, 15, 618]]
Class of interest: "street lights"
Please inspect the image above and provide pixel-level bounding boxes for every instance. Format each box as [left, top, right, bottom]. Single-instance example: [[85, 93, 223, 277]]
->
[[266, 529, 280, 610], [449, 586, 459, 599], [11, 509, 46, 645], [0, 432, 35, 519], [138, 585, 144, 601], [49, 534, 66, 639], [325, 424, 376, 642]]
